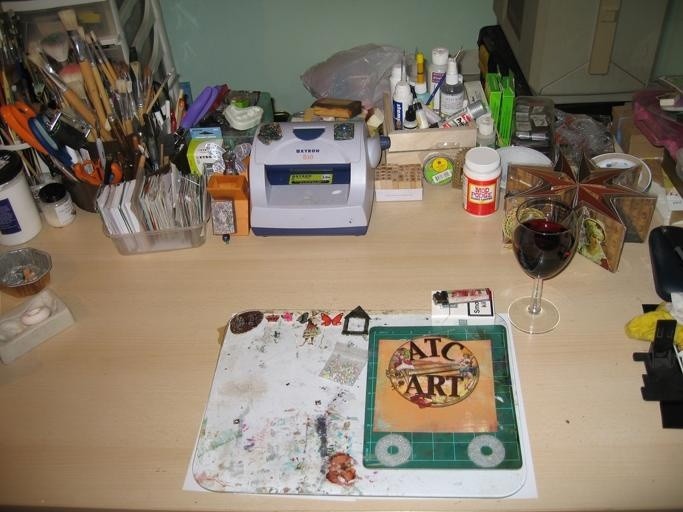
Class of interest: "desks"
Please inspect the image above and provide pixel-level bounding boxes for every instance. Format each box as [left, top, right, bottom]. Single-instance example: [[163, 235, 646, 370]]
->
[[0, 159, 683, 510]]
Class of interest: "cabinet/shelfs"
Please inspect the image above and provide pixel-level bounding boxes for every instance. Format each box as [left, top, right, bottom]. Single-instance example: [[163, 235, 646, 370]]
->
[[0, 1, 179, 106]]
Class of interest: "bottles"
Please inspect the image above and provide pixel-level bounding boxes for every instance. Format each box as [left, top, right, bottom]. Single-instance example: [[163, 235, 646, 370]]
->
[[0, 149, 40, 249], [388, 47, 449, 130], [37, 184, 76, 228], [461, 146, 502, 215]]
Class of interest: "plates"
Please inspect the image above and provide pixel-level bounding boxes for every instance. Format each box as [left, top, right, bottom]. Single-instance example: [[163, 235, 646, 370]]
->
[[496, 146, 554, 189], [590, 152, 652, 195]]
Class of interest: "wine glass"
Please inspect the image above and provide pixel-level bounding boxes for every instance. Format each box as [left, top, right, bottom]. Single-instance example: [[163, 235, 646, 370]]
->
[[507, 197, 579, 336]]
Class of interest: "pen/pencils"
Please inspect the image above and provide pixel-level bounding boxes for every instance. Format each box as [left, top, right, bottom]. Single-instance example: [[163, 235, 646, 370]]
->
[[6, 34, 185, 187]]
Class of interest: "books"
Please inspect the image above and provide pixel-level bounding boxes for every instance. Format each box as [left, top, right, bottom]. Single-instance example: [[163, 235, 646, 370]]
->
[[94, 161, 211, 255]]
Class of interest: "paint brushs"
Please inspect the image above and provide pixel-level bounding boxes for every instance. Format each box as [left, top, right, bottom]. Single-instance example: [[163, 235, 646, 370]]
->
[[426, 47, 464, 107]]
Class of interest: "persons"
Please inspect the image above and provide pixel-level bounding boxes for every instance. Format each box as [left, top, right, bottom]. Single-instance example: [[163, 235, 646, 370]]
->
[[577, 217, 611, 271]]
